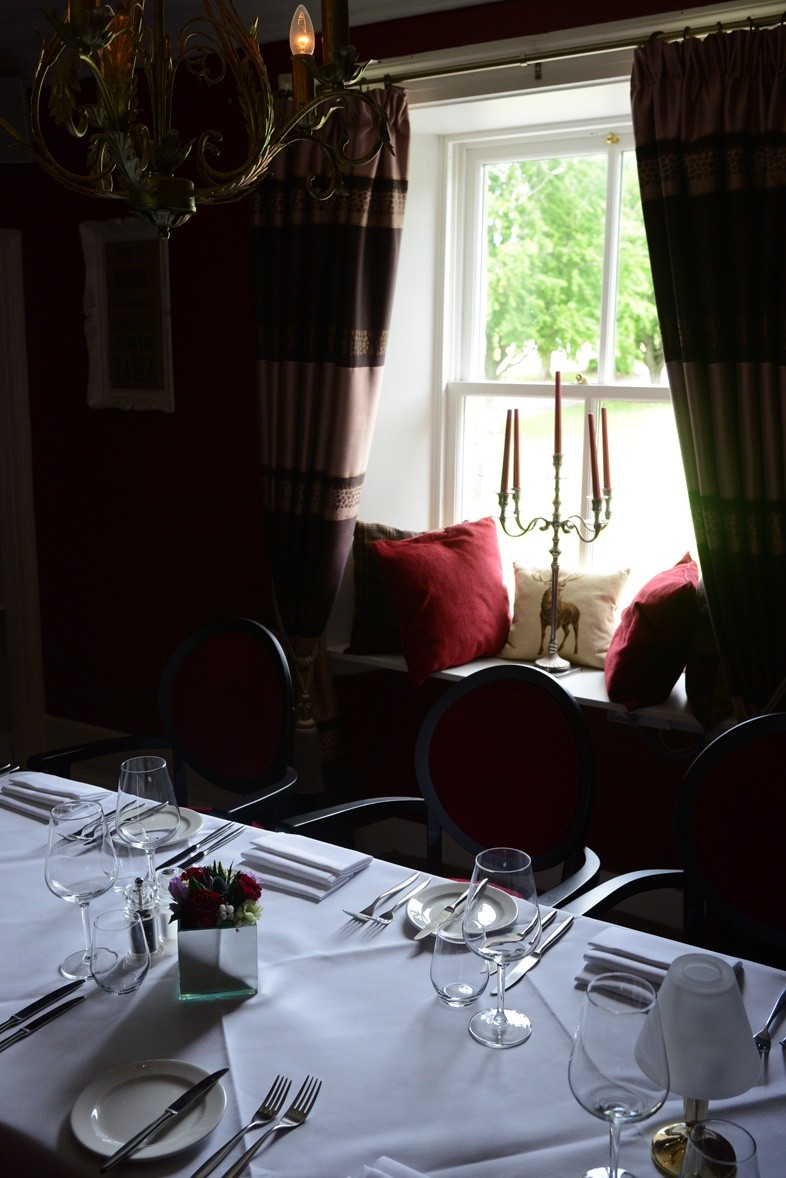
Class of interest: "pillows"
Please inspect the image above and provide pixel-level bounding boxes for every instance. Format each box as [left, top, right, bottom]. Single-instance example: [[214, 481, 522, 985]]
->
[[345, 523, 414, 656], [371, 522, 511, 683], [685, 588, 735, 736], [499, 595, 601, 669], [605, 579, 695, 711]]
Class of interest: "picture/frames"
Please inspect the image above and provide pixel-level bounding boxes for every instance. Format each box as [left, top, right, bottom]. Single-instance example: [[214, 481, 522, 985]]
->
[[76, 216, 177, 410]]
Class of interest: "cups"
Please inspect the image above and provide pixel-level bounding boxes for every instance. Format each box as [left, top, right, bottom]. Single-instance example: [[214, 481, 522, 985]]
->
[[90, 909, 151, 996], [101, 821, 151, 895], [680, 1119, 761, 1178], [431, 919, 489, 1007]]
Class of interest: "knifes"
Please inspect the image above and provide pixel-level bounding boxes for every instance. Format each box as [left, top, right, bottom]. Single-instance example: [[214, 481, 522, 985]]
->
[[100, 1066, 229, 1173], [413, 878, 574, 996], [82, 800, 170, 846], [0, 978, 86, 1051], [155, 820, 247, 875]]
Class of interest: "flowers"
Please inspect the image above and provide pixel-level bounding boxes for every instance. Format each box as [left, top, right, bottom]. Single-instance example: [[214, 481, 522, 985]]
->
[[167, 859, 263, 930]]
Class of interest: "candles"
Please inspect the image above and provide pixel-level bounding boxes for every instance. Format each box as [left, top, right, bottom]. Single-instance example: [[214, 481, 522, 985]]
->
[[501, 410, 512, 491], [601, 408, 610, 488], [588, 413, 601, 498], [513, 409, 520, 489], [554, 371, 562, 454]]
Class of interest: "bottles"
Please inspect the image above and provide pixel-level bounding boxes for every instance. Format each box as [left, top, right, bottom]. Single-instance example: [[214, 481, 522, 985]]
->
[[154, 867, 186, 943], [123, 877, 164, 966]]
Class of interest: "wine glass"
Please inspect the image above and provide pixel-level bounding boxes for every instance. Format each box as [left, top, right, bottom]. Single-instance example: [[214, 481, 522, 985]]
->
[[462, 848, 543, 1049], [567, 971, 670, 1178], [44, 799, 119, 980], [114, 755, 180, 883]]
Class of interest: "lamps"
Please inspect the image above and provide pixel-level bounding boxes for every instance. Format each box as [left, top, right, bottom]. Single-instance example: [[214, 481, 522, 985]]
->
[[2, 1, 393, 245]]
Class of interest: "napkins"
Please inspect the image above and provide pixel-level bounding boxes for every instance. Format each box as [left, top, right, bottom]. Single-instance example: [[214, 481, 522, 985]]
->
[[575, 924, 743, 1005], [234, 832, 373, 901], [0, 770, 112, 822]]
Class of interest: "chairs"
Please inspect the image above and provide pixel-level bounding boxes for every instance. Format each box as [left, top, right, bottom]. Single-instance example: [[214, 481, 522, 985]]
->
[[270, 664, 601, 909], [561, 711, 786, 969], [26, 617, 298, 826]]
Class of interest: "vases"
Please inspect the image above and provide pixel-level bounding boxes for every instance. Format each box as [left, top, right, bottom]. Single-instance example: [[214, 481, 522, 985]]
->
[[176, 915, 259, 1000]]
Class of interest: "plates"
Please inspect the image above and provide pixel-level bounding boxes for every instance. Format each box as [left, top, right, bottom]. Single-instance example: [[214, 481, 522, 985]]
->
[[107, 805, 203, 848], [70, 1059, 226, 1162]]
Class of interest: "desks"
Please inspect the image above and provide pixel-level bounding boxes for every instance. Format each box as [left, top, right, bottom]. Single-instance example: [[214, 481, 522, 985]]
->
[[0, 770, 786, 1178]]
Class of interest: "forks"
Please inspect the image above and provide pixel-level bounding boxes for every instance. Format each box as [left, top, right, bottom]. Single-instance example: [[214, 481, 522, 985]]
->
[[189, 1074, 322, 1178], [56, 800, 145, 842], [342, 871, 433, 925], [753, 989, 786, 1054]]
